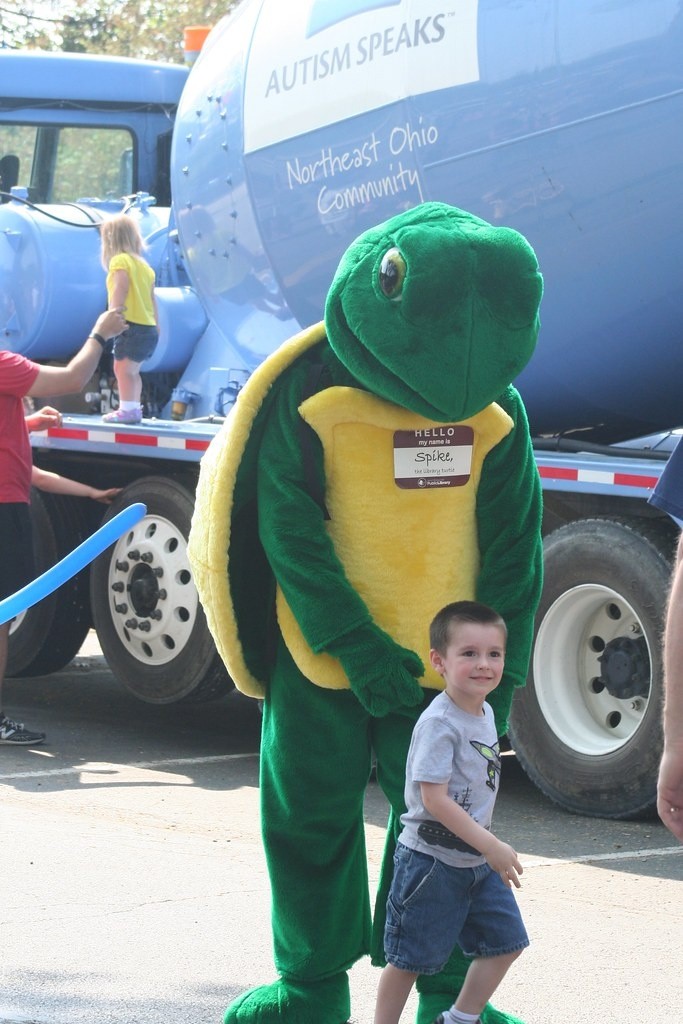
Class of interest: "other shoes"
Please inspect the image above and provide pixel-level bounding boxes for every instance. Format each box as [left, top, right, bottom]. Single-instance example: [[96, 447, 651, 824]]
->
[[434, 1014, 482, 1024]]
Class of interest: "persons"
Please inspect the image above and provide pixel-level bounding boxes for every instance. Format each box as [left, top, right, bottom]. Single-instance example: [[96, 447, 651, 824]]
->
[[645, 435, 682, 840], [98, 220, 160, 423], [262, 600, 531, 1024], [0, 307, 132, 745]]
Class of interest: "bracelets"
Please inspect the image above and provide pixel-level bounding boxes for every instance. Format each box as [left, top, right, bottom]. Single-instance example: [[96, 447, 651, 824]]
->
[[87, 332, 108, 349]]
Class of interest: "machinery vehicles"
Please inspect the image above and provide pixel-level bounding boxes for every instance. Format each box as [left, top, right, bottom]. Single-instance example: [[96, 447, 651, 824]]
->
[[1, 0, 683, 823]]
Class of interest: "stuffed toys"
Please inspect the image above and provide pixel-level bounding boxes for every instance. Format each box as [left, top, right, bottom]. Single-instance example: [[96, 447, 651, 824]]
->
[[187, 201, 544, 1024]]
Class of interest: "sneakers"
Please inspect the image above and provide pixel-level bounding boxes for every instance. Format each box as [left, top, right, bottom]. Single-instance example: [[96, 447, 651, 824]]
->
[[102, 407, 142, 424], [0, 712, 45, 745]]
